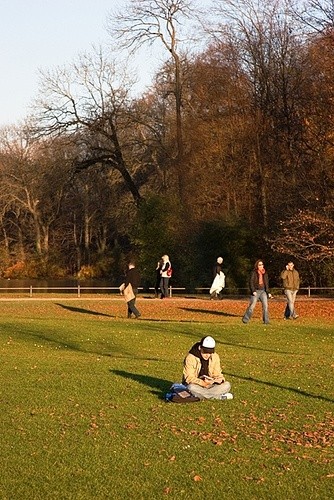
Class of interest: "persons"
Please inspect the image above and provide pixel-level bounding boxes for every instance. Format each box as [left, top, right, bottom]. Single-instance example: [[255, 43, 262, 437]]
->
[[153, 254, 172, 299], [180, 334, 233, 401], [241, 258, 273, 324], [119, 262, 142, 319], [209, 257, 226, 301], [280, 261, 300, 320]]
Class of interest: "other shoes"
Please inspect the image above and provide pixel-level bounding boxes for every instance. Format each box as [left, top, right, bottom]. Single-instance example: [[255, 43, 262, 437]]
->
[[220, 392, 233, 401]]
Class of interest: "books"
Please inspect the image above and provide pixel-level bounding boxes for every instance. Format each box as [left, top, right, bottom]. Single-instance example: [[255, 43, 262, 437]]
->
[[198, 375, 214, 381]]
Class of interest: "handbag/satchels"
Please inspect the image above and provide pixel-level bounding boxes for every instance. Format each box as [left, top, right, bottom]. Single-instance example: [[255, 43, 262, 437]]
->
[[165, 383, 201, 403], [167, 269, 173, 276]]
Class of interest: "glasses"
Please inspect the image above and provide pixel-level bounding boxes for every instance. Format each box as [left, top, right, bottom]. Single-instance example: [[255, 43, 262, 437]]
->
[[259, 264, 263, 266]]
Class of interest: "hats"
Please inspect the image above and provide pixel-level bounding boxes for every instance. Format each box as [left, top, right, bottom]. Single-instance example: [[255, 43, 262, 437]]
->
[[199, 335, 215, 353]]
